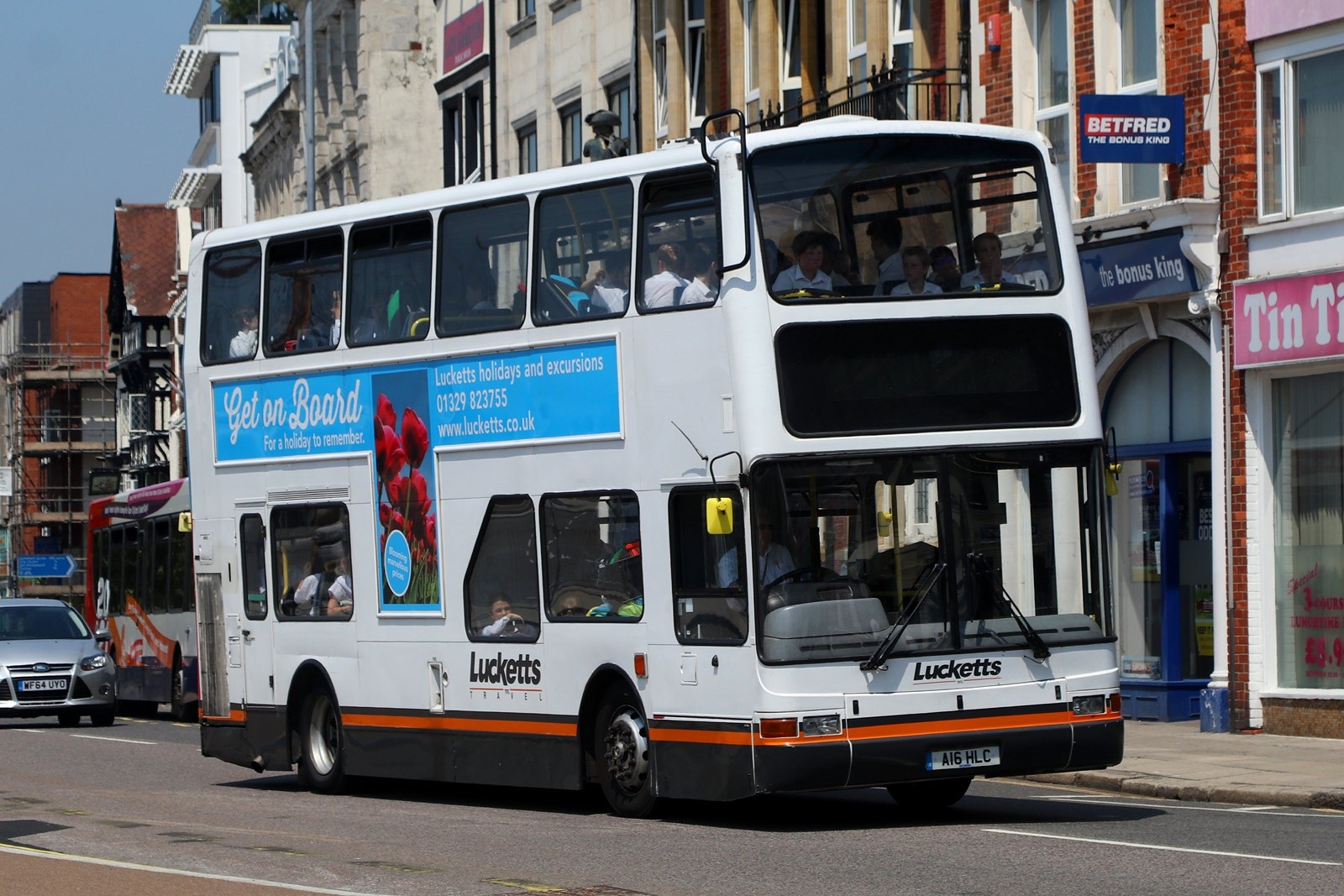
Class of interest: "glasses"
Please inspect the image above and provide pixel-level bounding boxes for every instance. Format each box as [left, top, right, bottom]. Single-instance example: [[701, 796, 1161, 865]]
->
[[758, 524, 771, 530]]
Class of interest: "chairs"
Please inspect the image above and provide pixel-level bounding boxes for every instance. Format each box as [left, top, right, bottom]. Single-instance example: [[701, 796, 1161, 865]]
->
[[294, 274, 605, 350], [272, 573, 635, 641]]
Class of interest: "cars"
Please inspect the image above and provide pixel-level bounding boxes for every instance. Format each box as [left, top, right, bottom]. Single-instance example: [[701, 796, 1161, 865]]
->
[[0, 596, 120, 730]]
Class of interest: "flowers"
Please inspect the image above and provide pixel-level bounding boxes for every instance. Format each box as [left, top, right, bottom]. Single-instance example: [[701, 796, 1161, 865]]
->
[[374, 395, 438, 610]]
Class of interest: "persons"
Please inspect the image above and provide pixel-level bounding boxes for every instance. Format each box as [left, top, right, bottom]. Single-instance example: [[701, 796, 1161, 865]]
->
[[228, 212, 1030, 363], [474, 592, 538, 643], [716, 509, 802, 612], [586, 565, 644, 618], [290, 552, 353, 620]]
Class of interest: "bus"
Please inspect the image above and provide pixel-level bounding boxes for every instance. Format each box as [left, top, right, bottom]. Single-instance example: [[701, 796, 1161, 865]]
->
[[178, 109, 1128, 824], [82, 475, 202, 724]]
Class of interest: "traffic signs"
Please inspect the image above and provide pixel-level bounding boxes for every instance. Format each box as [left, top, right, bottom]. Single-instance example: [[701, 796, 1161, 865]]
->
[[16, 553, 78, 578]]
